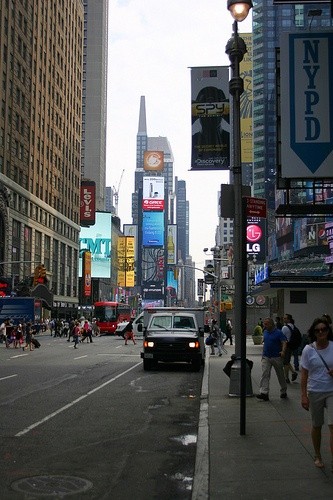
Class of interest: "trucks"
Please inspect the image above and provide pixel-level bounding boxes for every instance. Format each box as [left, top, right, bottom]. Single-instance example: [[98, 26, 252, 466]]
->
[[114, 306, 209, 340]]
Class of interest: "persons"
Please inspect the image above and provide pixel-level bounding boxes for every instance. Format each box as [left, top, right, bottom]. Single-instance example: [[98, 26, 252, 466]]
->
[[275, 314, 333, 383], [301, 318, 333, 467], [44, 316, 100, 349], [210, 318, 228, 356], [0, 318, 33, 351], [125, 321, 136, 345], [223, 317, 233, 345], [255, 318, 287, 401]]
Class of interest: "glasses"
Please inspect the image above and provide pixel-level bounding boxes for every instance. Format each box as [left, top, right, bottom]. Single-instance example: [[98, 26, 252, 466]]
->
[[313, 327, 328, 334]]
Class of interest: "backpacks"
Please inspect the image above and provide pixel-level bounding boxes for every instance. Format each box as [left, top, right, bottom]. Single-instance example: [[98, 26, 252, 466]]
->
[[285, 324, 302, 347]]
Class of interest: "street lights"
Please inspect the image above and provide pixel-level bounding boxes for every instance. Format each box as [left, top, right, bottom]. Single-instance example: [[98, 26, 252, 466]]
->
[[202, 245, 229, 361], [221, 0, 253, 401]]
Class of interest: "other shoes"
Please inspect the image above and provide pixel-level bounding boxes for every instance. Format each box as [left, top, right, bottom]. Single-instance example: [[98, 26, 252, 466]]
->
[[210, 353, 215, 355], [280, 393, 287, 398], [287, 379, 290, 383], [256, 393, 269, 401], [219, 354, 222, 356], [313, 454, 324, 467], [292, 373, 298, 381]]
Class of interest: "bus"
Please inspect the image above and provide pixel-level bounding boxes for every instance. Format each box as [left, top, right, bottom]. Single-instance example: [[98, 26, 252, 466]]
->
[[91, 301, 131, 335]]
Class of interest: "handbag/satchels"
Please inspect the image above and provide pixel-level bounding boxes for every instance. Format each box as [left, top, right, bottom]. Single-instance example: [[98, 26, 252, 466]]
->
[[206, 333, 214, 345]]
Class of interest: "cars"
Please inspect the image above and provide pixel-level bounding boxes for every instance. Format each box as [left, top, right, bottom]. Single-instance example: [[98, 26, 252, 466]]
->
[[137, 313, 209, 373]]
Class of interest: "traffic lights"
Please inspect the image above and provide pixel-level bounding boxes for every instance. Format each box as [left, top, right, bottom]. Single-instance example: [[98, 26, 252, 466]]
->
[[41, 268, 46, 278], [33, 276, 38, 285]]
